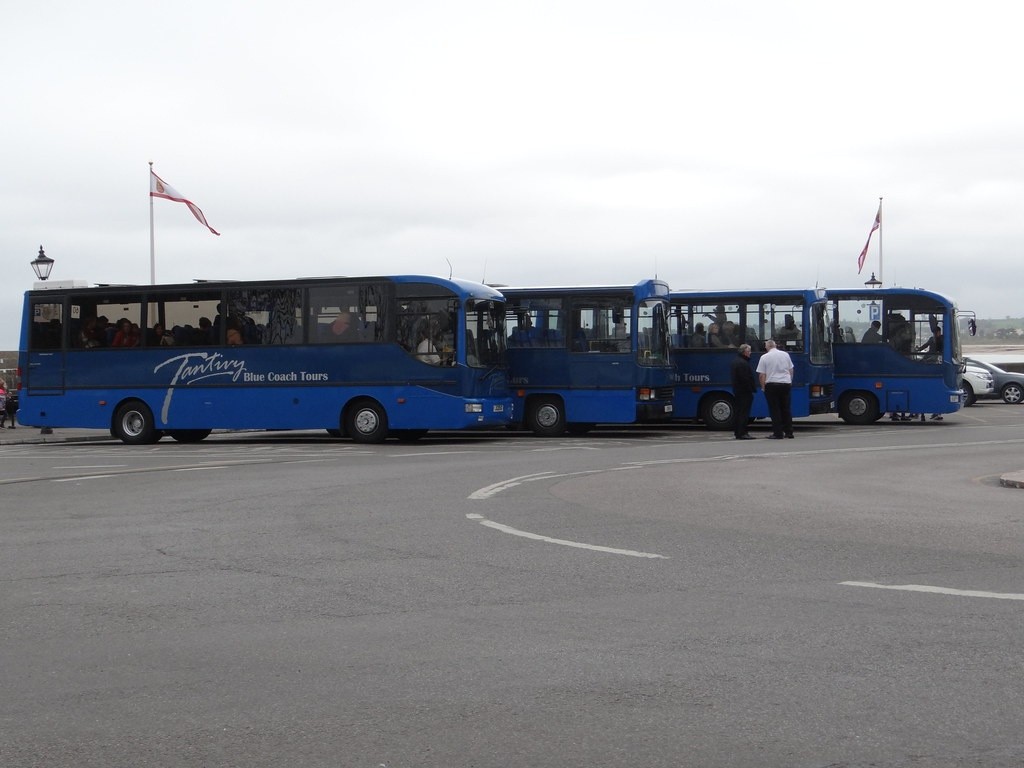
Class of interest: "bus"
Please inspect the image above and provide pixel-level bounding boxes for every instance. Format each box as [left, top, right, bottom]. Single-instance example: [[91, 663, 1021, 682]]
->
[[17, 275, 513, 442], [669, 287, 836, 427], [535, 289, 975, 440], [265, 281, 672, 436]]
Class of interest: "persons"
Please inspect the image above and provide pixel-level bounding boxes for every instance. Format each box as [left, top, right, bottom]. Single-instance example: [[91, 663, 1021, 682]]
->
[[396, 306, 458, 365], [916, 326, 944, 361], [861, 321, 883, 344], [729, 344, 757, 440], [778, 314, 801, 350], [682, 306, 759, 347], [756, 340, 794, 439], [0, 392, 15, 428], [889, 412, 944, 422], [72, 302, 361, 347]]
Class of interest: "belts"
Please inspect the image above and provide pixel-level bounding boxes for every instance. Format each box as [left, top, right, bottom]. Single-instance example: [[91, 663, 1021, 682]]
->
[[767, 383, 790, 385]]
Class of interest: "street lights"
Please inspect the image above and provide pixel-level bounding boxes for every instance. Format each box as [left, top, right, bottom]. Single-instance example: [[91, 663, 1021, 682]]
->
[[31, 245, 58, 434]]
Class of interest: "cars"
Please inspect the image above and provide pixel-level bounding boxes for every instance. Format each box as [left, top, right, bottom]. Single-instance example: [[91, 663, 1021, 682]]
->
[[962, 365, 995, 406], [963, 354, 1024, 404]]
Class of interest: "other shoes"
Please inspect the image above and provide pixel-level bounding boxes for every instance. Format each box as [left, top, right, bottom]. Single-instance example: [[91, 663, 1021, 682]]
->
[[740, 434, 756, 439], [929, 414, 938, 419], [915, 415, 918, 419], [901, 417, 911, 421], [909, 415, 915, 417], [8, 426, 16, 429], [921, 416, 925, 421], [735, 435, 741, 439], [934, 417, 943, 420], [784, 434, 794, 439], [892, 418, 899, 421], [0, 425, 5, 430], [768, 434, 783, 439]]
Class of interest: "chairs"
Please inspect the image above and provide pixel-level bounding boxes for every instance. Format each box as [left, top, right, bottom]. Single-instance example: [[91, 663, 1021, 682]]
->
[[33, 318, 208, 346], [636, 330, 761, 348], [512, 325, 592, 351], [839, 326, 856, 342]]
